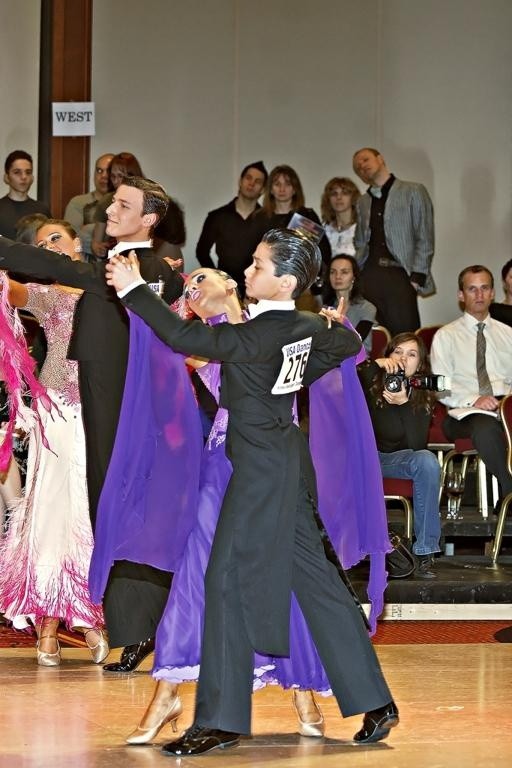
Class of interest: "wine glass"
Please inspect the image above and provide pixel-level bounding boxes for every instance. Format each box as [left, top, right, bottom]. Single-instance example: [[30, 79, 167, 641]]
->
[[444, 471, 466, 521]]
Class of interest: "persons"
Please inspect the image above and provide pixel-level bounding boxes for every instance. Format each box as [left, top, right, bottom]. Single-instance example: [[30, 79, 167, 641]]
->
[[91, 153, 186, 261], [2, 455, 22, 532], [64, 153, 115, 260], [106, 229, 398, 756], [353, 147, 437, 339], [196, 161, 268, 302], [360, 333, 442, 578], [430, 265, 512, 516], [320, 177, 362, 256], [489, 259, 512, 326], [319, 254, 378, 352], [1, 176, 183, 673], [106, 246, 344, 746], [1, 219, 110, 668], [239, 166, 332, 305], [2, 150, 52, 242]]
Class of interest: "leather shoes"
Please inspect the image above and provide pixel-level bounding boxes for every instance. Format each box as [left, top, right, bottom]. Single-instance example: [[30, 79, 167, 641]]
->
[[161, 724, 239, 757], [353, 701, 399, 743], [103, 635, 155, 674]]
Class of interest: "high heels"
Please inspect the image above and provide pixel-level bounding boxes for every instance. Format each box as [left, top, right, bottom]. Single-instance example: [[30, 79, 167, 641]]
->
[[291, 688, 326, 737], [125, 697, 182, 743], [36, 636, 62, 666], [84, 627, 110, 664]]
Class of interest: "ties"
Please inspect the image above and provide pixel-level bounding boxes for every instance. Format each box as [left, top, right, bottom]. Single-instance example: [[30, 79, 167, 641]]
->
[[476, 323, 492, 395]]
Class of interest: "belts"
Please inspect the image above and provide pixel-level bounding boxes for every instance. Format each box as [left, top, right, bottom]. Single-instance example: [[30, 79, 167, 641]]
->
[[377, 257, 401, 268]]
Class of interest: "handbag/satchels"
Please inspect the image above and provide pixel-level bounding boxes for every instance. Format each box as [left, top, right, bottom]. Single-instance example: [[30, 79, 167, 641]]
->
[[385, 528, 414, 579]]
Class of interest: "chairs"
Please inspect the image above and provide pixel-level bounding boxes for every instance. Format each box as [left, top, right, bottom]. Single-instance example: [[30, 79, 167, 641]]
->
[[369, 324, 512, 570]]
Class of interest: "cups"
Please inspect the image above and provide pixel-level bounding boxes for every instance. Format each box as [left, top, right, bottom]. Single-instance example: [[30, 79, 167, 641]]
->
[[443, 542, 456, 556]]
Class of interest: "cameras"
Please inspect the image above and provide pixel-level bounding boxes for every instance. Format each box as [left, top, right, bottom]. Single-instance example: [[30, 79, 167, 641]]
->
[[382, 370, 445, 407]]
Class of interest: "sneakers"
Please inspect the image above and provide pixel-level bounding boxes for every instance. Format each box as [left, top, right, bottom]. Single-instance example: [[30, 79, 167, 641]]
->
[[415, 555, 438, 578]]
